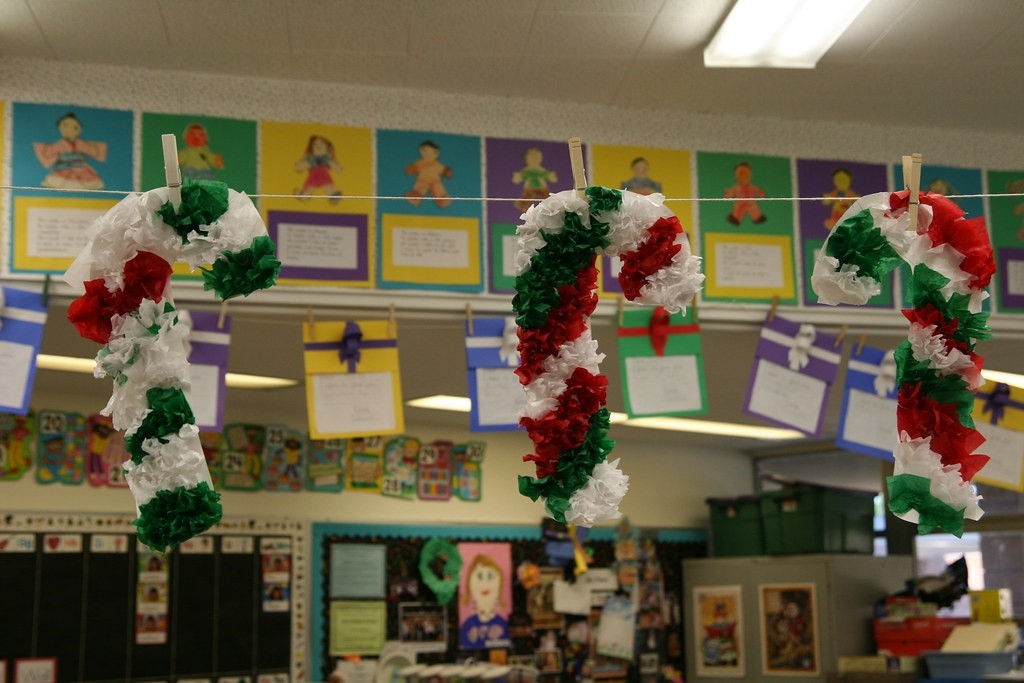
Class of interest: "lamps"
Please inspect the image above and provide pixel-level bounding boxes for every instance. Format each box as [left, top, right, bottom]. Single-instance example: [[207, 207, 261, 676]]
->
[[703, 0, 872, 69]]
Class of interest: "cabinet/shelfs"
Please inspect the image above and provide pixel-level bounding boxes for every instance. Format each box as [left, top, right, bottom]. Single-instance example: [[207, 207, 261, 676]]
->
[[681, 556, 914, 683]]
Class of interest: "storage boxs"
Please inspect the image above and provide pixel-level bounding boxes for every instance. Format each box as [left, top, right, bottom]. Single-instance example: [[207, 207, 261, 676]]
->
[[924, 651, 1021, 683], [873, 617, 969, 656], [707, 482, 878, 558]]
